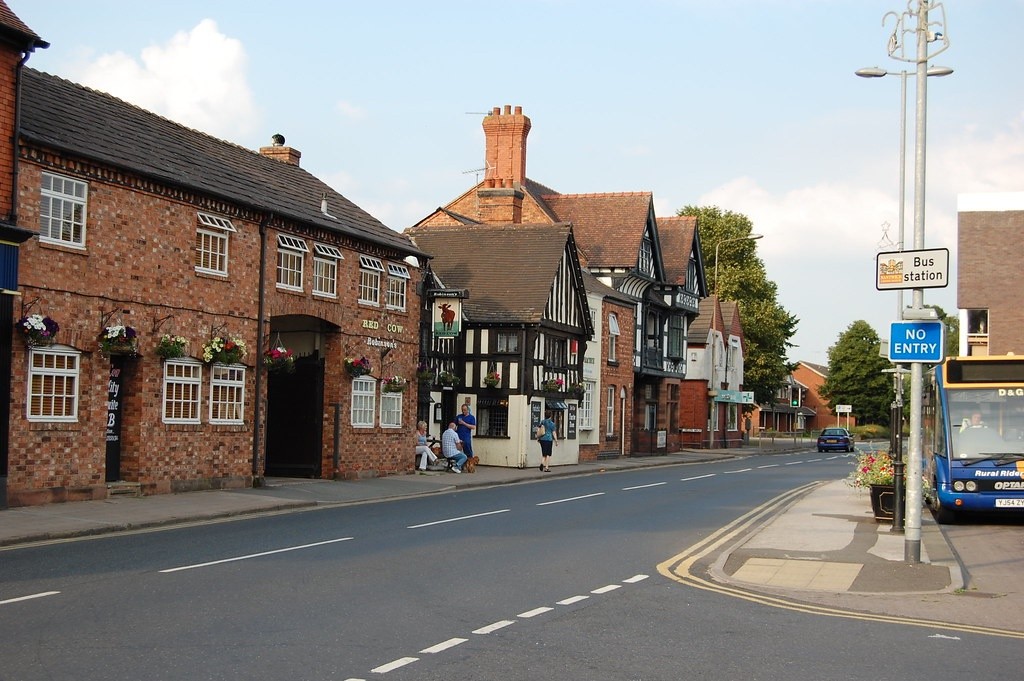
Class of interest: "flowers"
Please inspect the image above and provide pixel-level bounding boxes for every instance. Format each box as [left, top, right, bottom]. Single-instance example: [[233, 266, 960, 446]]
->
[[201, 336, 247, 366], [541, 379, 563, 395], [343, 356, 371, 379], [97, 326, 143, 361], [263, 349, 297, 374], [568, 381, 586, 395], [440, 371, 460, 387], [482, 371, 500, 385], [155, 333, 187, 360], [417, 365, 438, 387], [15, 313, 60, 353], [840, 440, 937, 510], [383, 375, 409, 395]]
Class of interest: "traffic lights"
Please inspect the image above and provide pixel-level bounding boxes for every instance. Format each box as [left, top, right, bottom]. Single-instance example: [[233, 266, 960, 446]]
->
[[801, 386, 807, 401], [790, 385, 801, 407]]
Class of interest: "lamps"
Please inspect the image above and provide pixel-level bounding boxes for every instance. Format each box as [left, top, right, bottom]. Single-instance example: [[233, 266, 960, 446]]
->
[[383, 256, 420, 268]]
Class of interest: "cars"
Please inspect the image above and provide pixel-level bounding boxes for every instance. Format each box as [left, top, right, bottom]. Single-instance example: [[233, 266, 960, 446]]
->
[[817, 428, 854, 453]]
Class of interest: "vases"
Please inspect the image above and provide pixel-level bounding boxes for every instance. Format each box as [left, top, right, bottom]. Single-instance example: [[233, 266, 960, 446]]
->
[[484, 378, 499, 386], [442, 379, 452, 387], [389, 385, 402, 391], [867, 484, 906, 525], [571, 391, 582, 399], [544, 385, 559, 393]]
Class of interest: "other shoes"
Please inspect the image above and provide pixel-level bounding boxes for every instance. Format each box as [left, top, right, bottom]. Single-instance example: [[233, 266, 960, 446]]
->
[[544, 469, 551, 472], [452, 466, 461, 473], [434, 459, 440, 466], [540, 464, 543, 471], [420, 469, 426, 475]]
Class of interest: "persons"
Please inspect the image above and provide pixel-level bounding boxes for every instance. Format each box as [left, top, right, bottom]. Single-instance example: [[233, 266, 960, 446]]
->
[[454, 404, 476, 458], [416, 421, 440, 471], [960, 410, 988, 435], [442, 423, 468, 474], [537, 411, 559, 472]]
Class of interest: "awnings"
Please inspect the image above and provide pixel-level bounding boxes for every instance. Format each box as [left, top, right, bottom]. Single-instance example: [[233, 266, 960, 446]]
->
[[546, 400, 569, 409]]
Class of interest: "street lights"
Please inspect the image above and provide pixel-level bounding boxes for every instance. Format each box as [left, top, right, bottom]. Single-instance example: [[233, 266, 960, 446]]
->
[[880, 367, 912, 534], [709, 234, 764, 450], [856, 66, 952, 321]]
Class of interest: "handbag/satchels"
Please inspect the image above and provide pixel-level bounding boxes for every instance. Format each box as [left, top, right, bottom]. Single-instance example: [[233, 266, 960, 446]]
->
[[537, 425, 545, 438]]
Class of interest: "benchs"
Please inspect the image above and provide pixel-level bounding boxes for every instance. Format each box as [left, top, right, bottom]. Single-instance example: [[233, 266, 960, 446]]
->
[[416, 439, 465, 471]]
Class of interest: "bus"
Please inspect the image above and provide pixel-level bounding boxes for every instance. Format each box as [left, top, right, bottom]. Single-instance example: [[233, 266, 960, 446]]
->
[[922, 352, 1024, 525]]
